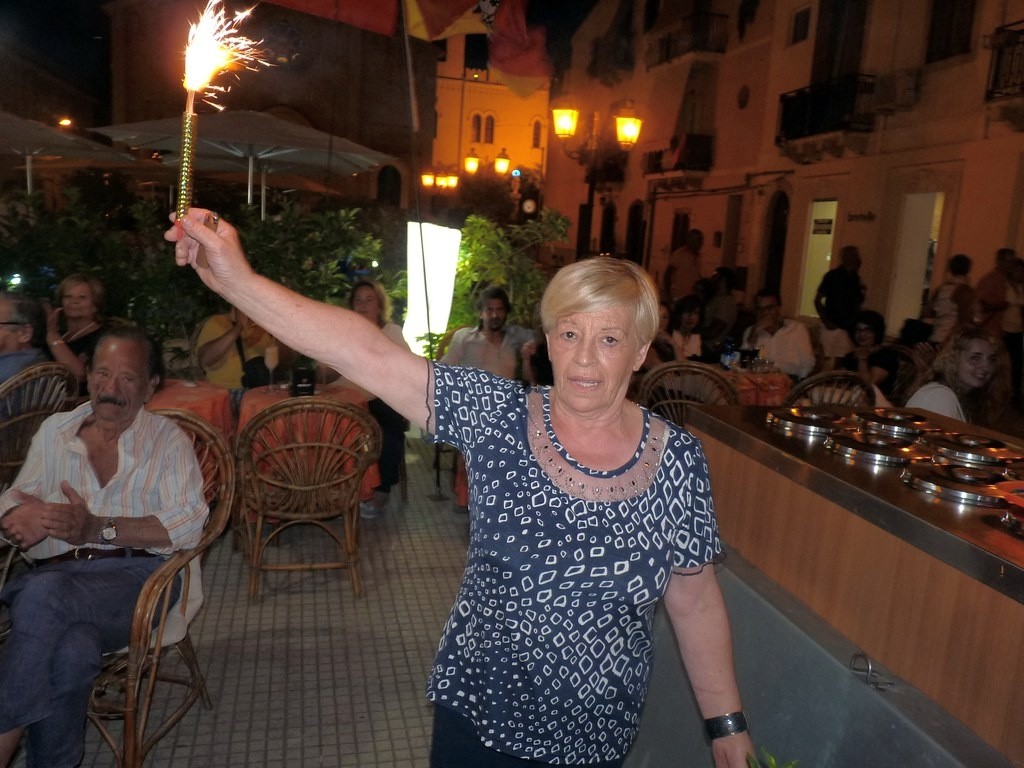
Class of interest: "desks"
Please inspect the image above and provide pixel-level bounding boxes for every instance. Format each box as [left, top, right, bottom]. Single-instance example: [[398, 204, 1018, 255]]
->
[[234, 384, 380, 523], [74, 379, 239, 500]]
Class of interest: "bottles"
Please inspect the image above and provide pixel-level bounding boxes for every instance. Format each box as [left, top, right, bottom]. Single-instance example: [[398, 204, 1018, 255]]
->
[[720, 326, 765, 373], [640, 129, 690, 172], [290, 354, 316, 397]]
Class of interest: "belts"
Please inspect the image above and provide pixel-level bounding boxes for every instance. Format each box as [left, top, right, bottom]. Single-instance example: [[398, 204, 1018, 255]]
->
[[34, 547, 156, 565]]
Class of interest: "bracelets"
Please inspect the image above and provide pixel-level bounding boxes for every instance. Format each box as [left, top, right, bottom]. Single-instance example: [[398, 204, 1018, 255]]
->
[[705, 712, 748, 740]]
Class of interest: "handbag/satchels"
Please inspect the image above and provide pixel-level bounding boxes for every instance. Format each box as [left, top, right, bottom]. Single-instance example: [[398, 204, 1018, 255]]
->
[[241, 357, 271, 388]]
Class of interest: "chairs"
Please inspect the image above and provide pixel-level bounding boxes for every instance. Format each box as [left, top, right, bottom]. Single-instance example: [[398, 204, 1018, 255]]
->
[[780, 370, 875, 408], [0, 323, 879, 768], [638, 358, 742, 427]]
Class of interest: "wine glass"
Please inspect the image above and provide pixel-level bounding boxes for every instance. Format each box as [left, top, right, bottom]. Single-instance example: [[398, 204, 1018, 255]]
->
[[262, 340, 280, 393]]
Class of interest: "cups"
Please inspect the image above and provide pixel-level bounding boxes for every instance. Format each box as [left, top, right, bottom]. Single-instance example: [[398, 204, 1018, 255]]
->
[[752, 357, 775, 372]]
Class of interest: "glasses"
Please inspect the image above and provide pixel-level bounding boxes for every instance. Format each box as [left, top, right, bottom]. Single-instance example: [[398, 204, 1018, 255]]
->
[[856, 327, 872, 333], [755, 304, 774, 314]]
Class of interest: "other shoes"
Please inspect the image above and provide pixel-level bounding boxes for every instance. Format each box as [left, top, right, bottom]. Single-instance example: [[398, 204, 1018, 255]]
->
[[453, 465, 469, 513], [360, 492, 388, 518]]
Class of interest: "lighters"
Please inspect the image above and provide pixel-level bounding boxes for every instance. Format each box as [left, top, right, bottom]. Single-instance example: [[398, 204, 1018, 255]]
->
[[196, 212, 220, 269]]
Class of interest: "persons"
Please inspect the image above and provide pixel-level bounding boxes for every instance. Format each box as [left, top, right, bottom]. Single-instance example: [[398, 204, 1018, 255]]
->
[[835, 312, 896, 385], [438, 288, 539, 385], [901, 330, 1013, 426], [922, 248, 1024, 415], [0, 321, 203, 768], [0, 464, 0, 466], [632, 267, 815, 394], [164, 208, 759, 768], [814, 245, 866, 342], [318, 278, 414, 529], [663, 230, 704, 307], [0, 272, 109, 411], [195, 304, 299, 416]]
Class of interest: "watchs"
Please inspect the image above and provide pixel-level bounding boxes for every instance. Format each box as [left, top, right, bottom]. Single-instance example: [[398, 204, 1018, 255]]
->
[[96, 518, 116, 544]]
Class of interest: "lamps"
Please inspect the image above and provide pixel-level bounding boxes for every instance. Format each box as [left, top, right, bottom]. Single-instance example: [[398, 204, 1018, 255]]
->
[[613, 99, 645, 160], [548, 90, 590, 166]]
